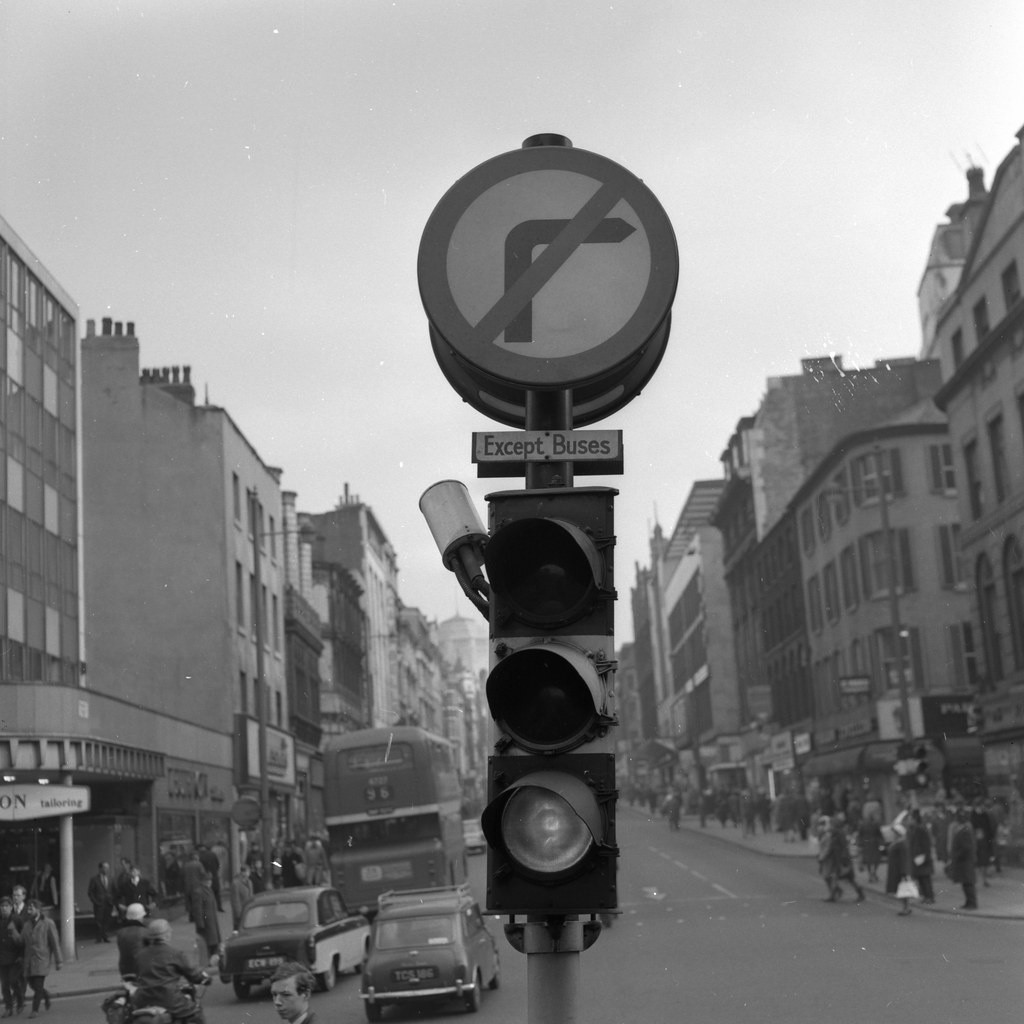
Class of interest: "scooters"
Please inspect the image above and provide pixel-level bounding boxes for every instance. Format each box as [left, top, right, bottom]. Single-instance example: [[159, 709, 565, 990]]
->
[[101, 952, 220, 1024]]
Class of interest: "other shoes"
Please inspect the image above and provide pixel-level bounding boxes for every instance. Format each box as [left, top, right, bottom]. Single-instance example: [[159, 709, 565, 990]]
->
[[16, 1001, 24, 1015], [837, 887, 844, 898], [822, 896, 838, 903], [3, 1009, 12, 1019], [921, 896, 934, 905], [855, 895, 865, 902], [29, 1010, 39, 1019], [44, 992, 51, 1010], [959, 901, 977, 911], [898, 909, 912, 917]]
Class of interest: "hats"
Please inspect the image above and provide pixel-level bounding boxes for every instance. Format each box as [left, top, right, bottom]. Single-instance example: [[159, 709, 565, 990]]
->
[[199, 872, 213, 881], [892, 824, 907, 838]]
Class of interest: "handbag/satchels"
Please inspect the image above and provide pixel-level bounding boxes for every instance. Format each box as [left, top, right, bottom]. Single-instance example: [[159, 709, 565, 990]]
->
[[895, 877, 920, 899]]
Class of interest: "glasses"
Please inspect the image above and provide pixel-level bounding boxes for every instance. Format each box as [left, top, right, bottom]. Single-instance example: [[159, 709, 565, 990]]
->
[[12, 894, 23, 897]]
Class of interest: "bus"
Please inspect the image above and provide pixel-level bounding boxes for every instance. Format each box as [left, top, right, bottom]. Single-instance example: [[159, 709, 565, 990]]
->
[[322, 729, 467, 920]]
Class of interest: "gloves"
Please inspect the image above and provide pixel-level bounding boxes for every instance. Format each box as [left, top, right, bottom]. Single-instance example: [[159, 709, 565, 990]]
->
[[196, 972, 211, 985]]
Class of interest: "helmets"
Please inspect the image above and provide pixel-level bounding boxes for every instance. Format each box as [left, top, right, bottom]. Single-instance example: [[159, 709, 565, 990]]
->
[[125, 902, 146, 920], [147, 919, 172, 944]]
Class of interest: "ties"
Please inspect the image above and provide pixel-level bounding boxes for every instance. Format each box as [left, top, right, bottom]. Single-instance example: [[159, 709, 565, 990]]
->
[[102, 874, 106, 887], [16, 903, 19, 914]]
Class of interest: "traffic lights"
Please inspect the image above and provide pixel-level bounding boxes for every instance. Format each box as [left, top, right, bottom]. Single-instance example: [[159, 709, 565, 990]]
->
[[480, 489, 623, 920], [900, 745, 929, 789]]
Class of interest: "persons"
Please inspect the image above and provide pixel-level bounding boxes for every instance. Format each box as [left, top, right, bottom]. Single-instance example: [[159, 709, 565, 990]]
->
[[0, 829, 334, 1024], [619, 780, 1024, 917]]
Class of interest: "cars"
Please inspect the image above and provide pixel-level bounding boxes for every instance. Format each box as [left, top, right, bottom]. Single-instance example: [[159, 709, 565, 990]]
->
[[360, 882, 500, 1023], [217, 884, 371, 1000], [463, 819, 486, 852]]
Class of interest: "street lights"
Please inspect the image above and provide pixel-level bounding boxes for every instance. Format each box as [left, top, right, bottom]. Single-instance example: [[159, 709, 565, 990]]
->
[[248, 488, 317, 888], [817, 447, 930, 811]]
